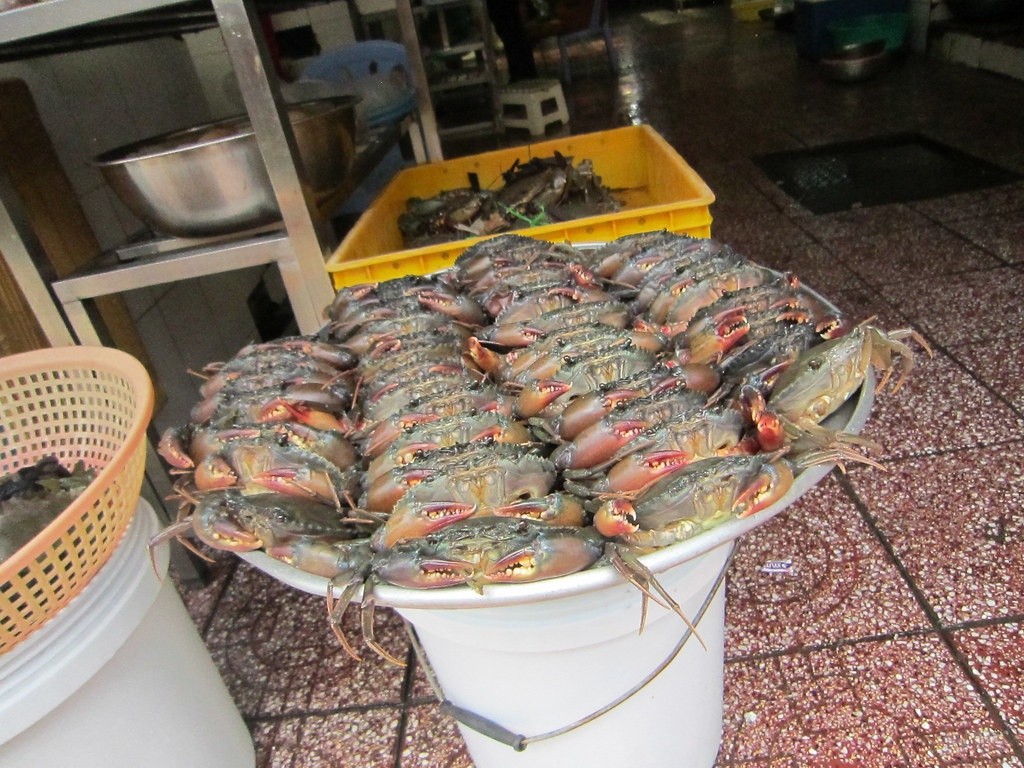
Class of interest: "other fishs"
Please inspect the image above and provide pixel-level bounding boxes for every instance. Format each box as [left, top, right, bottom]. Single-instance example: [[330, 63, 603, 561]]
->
[[0, 453, 113, 623], [397, 155, 621, 247]]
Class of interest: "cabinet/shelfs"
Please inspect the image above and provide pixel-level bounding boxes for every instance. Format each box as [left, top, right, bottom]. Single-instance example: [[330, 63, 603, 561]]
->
[[0, 0, 444, 589], [361, 0, 503, 141]]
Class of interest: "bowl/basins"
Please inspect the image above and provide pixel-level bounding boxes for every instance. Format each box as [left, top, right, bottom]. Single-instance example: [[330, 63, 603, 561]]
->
[[818, 44, 888, 81], [758, 7, 789, 19], [84, 95, 362, 238], [828, 12, 909, 51]]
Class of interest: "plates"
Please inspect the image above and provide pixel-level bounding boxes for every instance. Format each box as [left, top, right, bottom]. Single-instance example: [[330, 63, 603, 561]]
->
[[360, 88, 416, 127]]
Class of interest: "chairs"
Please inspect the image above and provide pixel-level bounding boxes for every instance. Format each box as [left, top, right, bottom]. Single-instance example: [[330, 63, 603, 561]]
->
[[537, 0, 618, 83]]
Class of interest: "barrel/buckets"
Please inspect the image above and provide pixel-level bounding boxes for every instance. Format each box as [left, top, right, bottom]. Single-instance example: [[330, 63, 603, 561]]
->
[[392, 536, 745, 767], [0, 499, 259, 768]]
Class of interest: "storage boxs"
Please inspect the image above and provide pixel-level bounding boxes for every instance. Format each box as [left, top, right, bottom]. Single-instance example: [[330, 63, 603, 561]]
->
[[325, 123, 715, 294]]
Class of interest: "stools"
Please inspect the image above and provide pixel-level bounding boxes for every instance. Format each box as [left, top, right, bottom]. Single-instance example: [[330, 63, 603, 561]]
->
[[499, 79, 569, 137]]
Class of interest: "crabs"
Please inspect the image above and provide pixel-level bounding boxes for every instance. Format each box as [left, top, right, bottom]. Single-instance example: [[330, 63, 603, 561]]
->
[[148, 227, 937, 668]]
